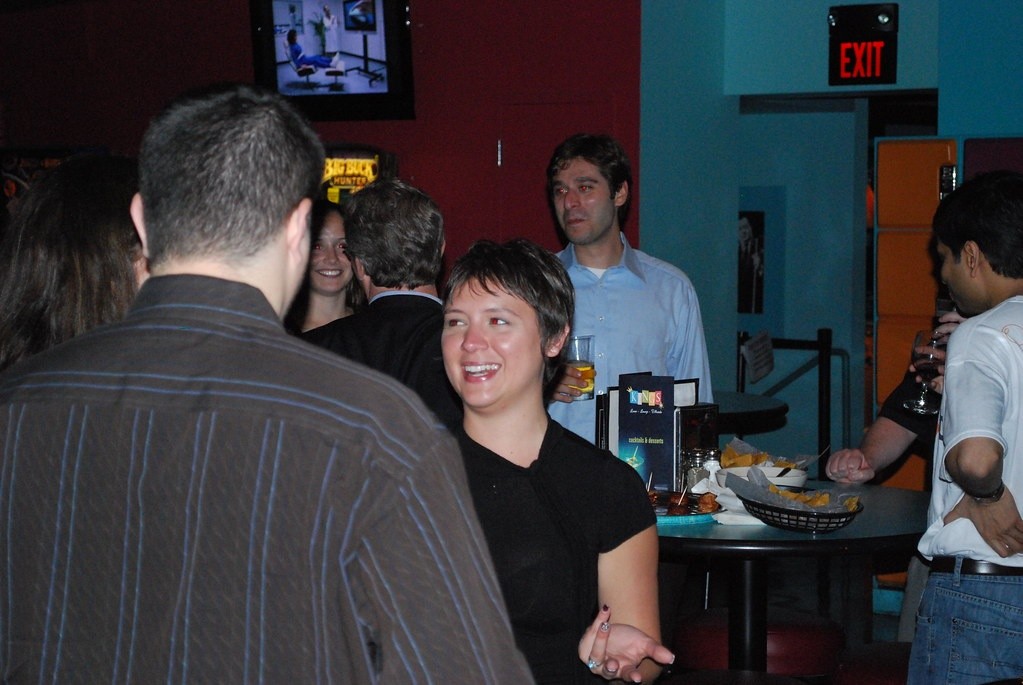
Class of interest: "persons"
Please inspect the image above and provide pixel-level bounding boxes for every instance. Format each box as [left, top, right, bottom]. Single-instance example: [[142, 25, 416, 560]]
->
[[546, 131, 715, 444], [288, 3, 296, 29], [0, 175, 463, 424], [287, 28, 339, 69], [0, 90, 537, 685], [323, 4, 338, 58], [825, 170, 1023, 685]]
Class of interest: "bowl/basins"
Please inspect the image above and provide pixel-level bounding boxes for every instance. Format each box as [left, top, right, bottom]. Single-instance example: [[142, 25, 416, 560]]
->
[[716, 466, 809, 493]]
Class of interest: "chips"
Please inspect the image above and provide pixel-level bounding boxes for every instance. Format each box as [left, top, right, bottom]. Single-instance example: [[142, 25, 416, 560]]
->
[[768, 484, 860, 512], [720, 443, 795, 470]]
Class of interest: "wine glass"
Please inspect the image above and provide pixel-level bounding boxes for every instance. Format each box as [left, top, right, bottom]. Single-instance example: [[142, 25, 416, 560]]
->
[[901, 328, 948, 416]]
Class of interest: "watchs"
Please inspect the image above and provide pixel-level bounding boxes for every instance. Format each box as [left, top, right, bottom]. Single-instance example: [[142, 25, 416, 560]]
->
[[966, 479, 1005, 504]]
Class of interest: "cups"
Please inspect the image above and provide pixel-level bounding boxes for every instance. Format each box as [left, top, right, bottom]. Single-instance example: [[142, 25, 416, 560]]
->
[[567, 334, 594, 400], [929, 298, 958, 348]]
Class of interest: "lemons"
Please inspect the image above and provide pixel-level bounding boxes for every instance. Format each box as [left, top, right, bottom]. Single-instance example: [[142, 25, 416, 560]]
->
[[574, 366, 591, 371]]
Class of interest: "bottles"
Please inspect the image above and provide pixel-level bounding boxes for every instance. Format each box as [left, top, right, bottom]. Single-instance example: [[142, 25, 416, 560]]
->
[[678, 447, 722, 494]]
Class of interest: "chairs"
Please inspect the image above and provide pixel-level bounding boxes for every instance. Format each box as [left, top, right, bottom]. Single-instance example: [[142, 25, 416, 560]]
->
[[283, 37, 319, 88]]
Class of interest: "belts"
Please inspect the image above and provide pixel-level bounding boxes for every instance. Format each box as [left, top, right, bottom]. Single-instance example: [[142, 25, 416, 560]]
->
[[927, 554, 1023, 575]]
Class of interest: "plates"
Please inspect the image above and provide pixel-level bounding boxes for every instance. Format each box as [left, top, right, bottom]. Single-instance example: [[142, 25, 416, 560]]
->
[[652, 490, 726, 525]]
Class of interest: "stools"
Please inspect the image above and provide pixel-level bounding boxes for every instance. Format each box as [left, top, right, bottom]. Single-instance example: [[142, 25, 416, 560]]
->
[[324, 60, 345, 91]]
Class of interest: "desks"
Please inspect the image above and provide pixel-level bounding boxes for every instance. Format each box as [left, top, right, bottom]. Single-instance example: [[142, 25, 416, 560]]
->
[[709, 389, 789, 442], [654, 479, 933, 675]]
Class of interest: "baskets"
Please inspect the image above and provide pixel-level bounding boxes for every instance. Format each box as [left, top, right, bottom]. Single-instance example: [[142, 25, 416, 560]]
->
[[736, 487, 864, 533]]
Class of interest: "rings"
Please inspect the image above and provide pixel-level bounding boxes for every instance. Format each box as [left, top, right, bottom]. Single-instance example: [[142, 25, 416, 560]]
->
[[438, 235, 676, 685], [586, 655, 605, 669], [1004, 545, 1010, 551]]
